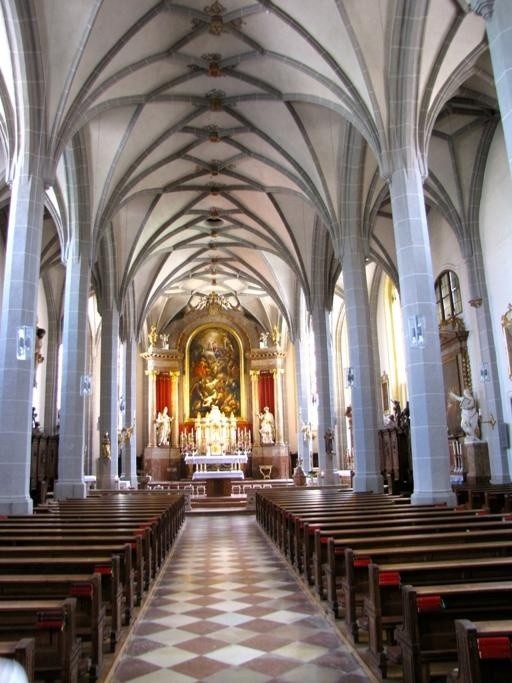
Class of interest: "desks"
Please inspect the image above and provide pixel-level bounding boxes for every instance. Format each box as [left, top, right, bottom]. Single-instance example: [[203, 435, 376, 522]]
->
[[184, 455, 248, 470], [193, 470, 244, 496]]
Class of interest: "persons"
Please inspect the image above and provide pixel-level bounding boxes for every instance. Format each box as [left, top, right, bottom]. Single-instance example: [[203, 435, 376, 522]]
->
[[450, 389, 480, 440], [256, 406, 275, 442], [188, 333, 240, 415], [155, 404, 175, 447]]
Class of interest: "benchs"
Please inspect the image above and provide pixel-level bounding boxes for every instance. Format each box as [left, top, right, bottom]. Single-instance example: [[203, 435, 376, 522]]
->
[[252, 487, 512, 682], [0, 487, 185, 683]]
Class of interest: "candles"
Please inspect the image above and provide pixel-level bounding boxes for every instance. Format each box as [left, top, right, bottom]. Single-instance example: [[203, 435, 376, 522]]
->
[[196, 463, 207, 471], [231, 463, 240, 470], [179, 427, 251, 445]]
